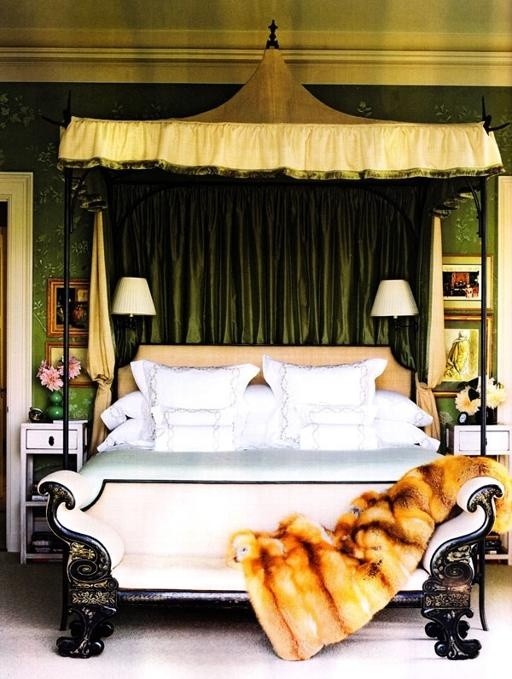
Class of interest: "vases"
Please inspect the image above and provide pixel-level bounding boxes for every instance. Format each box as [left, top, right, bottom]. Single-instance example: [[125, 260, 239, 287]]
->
[[46, 390, 65, 419], [476, 406, 493, 422]]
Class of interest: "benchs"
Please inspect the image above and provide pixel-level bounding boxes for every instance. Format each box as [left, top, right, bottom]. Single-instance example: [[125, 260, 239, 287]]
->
[[38, 469, 505, 658]]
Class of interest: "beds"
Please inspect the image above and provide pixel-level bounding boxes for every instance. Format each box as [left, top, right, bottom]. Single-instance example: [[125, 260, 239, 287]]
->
[[79, 343, 444, 480]]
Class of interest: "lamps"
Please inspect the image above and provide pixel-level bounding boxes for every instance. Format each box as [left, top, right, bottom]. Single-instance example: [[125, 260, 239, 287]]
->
[[110, 277, 157, 329], [370, 280, 420, 334]]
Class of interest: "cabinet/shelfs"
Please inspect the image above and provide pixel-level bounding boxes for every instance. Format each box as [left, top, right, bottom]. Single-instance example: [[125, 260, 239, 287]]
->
[[447, 423, 510, 456], [21, 420, 90, 564]]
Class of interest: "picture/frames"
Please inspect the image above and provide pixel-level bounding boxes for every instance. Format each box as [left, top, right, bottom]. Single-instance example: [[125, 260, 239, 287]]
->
[[433, 253, 494, 398], [46, 278, 99, 387]]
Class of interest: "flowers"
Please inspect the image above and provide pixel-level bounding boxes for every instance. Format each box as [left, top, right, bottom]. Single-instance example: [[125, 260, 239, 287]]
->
[[452, 375, 506, 416], [36, 355, 81, 390]]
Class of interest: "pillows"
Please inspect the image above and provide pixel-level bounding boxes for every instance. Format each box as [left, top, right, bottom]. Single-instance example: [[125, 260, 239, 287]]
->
[[128, 356, 389, 457]]
